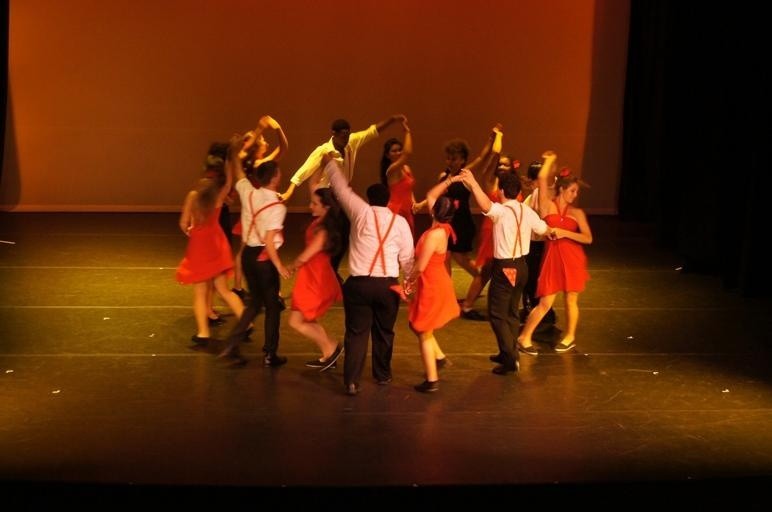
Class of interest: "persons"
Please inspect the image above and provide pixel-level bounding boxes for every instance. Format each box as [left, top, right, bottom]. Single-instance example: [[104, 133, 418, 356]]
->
[[174, 115, 593, 398]]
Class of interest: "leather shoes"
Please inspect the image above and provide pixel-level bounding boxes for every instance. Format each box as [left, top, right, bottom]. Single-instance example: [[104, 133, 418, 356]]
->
[[305, 358, 336, 368], [319, 343, 345, 372], [515, 339, 538, 356], [191, 286, 287, 368], [554, 337, 576, 352], [414, 380, 440, 393], [492, 365, 515, 376], [423, 357, 453, 378], [462, 309, 484, 321], [490, 354, 502, 363], [344, 381, 362, 396]]
